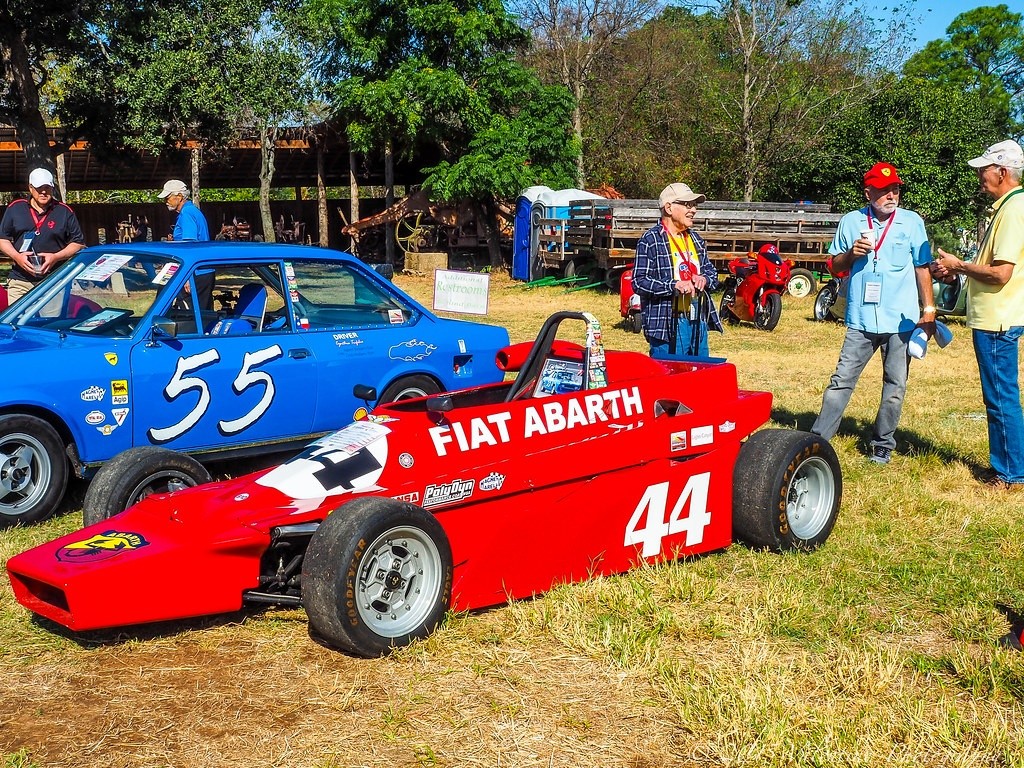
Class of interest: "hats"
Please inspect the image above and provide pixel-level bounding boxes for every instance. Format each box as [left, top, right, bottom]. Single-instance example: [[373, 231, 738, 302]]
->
[[156, 180, 190, 198], [863, 162, 904, 189], [29, 168, 54, 188], [656, 183, 706, 208], [967, 139, 1024, 169]]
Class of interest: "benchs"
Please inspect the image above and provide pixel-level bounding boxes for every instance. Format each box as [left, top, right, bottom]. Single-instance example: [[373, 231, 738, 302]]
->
[[305, 310, 389, 331]]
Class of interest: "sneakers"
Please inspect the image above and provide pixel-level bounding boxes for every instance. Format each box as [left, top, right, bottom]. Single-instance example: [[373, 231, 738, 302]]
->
[[976, 467, 1023, 494], [868, 442, 892, 464]]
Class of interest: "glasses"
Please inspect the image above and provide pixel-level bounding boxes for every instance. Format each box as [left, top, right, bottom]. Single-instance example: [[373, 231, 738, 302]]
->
[[672, 201, 698, 209]]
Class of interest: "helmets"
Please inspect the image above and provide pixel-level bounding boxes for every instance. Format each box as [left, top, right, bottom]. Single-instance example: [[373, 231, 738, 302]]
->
[[758, 244, 779, 255]]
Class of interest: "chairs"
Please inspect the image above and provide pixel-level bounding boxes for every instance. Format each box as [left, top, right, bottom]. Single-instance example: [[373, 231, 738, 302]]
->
[[504, 310, 609, 412], [204, 283, 269, 334]]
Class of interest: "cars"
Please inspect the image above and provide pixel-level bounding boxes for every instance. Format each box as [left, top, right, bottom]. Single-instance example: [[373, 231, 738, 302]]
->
[[0, 239, 508, 522], [0, 285, 102, 320]]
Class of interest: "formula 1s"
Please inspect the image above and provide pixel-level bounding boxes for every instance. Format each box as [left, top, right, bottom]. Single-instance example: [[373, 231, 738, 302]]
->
[[7, 310, 843, 657]]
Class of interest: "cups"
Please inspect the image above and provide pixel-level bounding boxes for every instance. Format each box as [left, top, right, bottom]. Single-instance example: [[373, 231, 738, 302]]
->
[[859, 229, 877, 252], [29, 254, 43, 276]]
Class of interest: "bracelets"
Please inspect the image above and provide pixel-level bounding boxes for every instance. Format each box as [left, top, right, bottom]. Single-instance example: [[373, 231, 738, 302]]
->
[[923, 306, 938, 313]]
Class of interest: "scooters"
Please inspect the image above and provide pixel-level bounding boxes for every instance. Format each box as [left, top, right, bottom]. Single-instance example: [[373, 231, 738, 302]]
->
[[612, 262, 645, 333]]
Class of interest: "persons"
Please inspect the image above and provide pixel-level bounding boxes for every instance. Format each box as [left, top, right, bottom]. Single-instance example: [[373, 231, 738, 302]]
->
[[128, 214, 156, 279], [0, 168, 85, 317], [632, 183, 722, 357], [157, 180, 215, 309], [810, 162, 937, 462], [929, 140, 1024, 494]]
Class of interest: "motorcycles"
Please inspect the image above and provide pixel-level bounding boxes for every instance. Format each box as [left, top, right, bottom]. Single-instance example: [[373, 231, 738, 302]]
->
[[918, 270, 968, 322], [718, 243, 794, 330], [814, 274, 843, 323]]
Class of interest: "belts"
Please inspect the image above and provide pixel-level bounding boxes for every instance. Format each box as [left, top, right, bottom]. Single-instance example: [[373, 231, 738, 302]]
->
[[677, 309, 706, 319]]
